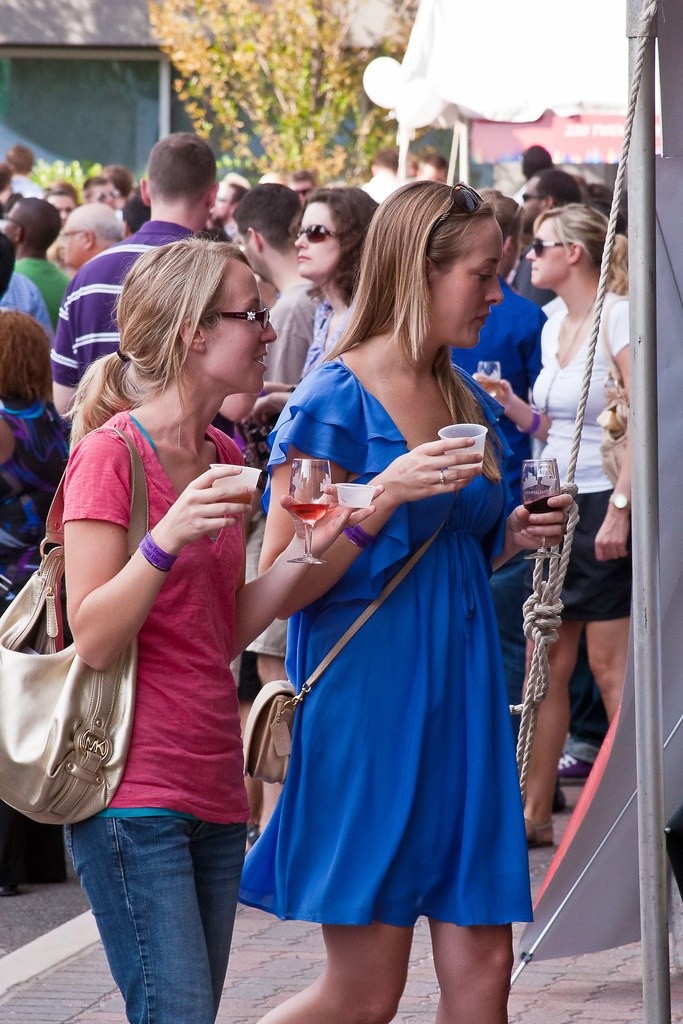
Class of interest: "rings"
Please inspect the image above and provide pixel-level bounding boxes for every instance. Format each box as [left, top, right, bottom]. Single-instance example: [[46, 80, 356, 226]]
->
[[439, 470, 447, 483]]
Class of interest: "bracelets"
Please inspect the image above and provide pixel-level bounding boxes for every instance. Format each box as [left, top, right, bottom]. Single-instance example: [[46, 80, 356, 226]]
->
[[143, 553, 167, 573], [517, 407, 541, 435], [342, 524, 376, 550], [135, 531, 178, 571], [260, 389, 265, 396]]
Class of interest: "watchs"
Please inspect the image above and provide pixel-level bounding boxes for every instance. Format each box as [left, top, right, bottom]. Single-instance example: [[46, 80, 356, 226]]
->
[[609, 492, 631, 510]]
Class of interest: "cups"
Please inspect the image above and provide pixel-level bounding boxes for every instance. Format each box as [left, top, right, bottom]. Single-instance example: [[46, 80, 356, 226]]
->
[[209, 463, 263, 516], [336, 482, 376, 509], [476, 361, 501, 398], [438, 423, 488, 470]]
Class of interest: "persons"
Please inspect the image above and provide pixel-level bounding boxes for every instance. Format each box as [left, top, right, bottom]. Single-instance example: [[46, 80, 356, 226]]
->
[[63, 234, 386, 1024], [238, 182, 574, 1024], [0, 135, 631, 895]]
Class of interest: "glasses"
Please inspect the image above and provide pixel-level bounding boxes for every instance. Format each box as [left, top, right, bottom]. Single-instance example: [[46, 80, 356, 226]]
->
[[429, 180, 485, 238], [296, 224, 339, 244], [1, 213, 23, 231], [532, 238, 574, 257], [216, 308, 270, 330], [58, 228, 84, 244], [292, 187, 311, 199], [96, 189, 119, 203], [522, 193, 545, 203]]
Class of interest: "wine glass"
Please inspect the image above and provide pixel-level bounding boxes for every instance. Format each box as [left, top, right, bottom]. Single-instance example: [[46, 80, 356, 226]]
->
[[520, 458, 564, 559], [286, 458, 332, 563]]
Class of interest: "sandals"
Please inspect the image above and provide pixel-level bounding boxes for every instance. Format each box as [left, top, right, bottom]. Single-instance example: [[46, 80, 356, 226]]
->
[[524, 812, 554, 850]]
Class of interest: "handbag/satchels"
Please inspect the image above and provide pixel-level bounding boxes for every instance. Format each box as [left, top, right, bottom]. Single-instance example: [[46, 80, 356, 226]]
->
[[0, 427, 148, 826], [241, 680, 296, 785]]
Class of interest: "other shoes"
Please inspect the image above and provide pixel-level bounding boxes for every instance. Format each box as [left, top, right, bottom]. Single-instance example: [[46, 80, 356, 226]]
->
[[557, 753, 594, 778], [246, 824, 261, 846], [0, 883, 18, 896], [552, 791, 566, 815]]
